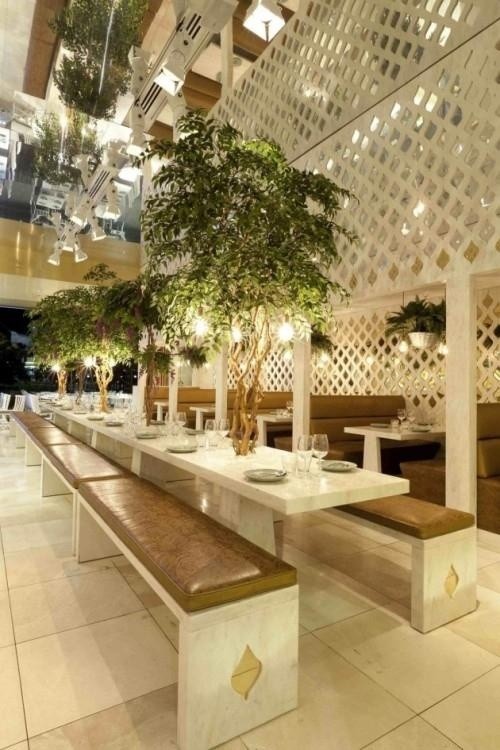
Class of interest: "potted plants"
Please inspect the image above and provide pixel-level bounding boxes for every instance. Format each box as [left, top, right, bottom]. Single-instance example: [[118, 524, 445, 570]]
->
[[384, 294, 445, 349]]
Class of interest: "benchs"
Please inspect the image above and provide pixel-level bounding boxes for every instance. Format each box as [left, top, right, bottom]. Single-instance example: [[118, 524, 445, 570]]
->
[[399, 403, 500, 535], [334, 496, 478, 635], [145, 387, 406, 461], [40, 444, 133, 556], [10, 411, 54, 462], [25, 427, 81, 493], [75, 477, 298, 750]]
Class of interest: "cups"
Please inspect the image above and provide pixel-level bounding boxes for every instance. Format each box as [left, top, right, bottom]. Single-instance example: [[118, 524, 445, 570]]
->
[[176, 411, 187, 427], [391, 408, 409, 430]]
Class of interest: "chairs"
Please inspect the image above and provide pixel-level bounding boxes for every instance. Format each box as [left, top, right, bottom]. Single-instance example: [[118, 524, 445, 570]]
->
[[0, 389, 49, 423]]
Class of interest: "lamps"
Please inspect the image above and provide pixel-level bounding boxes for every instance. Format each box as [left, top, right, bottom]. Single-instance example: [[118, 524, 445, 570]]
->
[[126, 104, 147, 156], [153, 27, 192, 96], [70, 198, 94, 227], [242, 1, 285, 43], [102, 183, 120, 221], [87, 210, 106, 242], [73, 236, 89, 263], [48, 238, 66, 266]]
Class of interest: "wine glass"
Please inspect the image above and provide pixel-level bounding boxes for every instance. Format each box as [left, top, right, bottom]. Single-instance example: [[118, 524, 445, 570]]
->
[[295, 433, 330, 479], [204, 418, 231, 453]]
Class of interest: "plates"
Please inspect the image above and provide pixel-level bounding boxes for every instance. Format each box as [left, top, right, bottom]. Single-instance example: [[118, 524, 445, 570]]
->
[[370, 422, 390, 429], [167, 445, 197, 454], [105, 421, 122, 426], [136, 433, 157, 439], [243, 469, 289, 482], [411, 425, 431, 432], [317, 460, 358, 472], [88, 416, 104, 420]]
